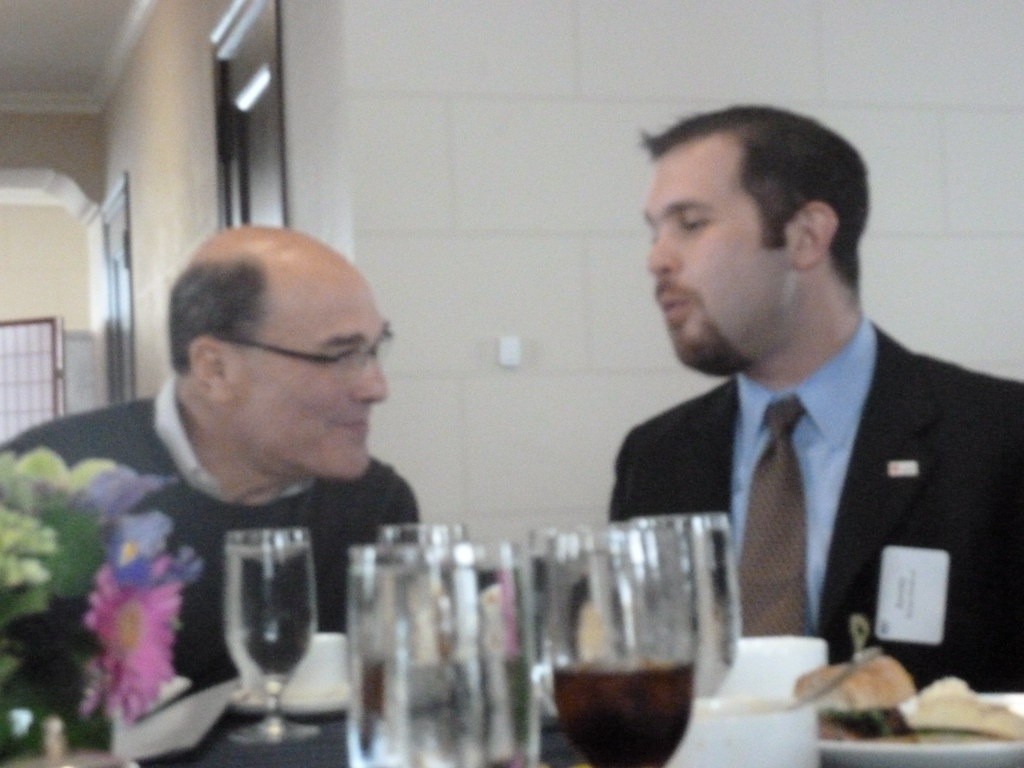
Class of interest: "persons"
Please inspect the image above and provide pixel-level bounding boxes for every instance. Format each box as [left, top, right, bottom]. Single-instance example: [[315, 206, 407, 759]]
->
[[614, 106, 1024, 692], [0, 228, 419, 692]]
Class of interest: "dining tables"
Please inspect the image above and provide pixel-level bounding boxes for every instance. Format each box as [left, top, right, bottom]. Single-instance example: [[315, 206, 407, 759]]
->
[[161, 653, 601, 768]]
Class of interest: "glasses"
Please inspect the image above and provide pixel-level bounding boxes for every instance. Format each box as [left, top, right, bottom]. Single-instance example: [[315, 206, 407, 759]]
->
[[219, 331, 392, 374]]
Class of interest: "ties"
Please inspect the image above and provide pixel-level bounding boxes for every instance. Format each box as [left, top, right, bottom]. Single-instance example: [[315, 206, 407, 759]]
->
[[738, 396, 808, 636]]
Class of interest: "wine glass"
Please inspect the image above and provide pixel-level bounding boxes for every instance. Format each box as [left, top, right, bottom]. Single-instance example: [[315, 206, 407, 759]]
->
[[225, 525, 322, 746]]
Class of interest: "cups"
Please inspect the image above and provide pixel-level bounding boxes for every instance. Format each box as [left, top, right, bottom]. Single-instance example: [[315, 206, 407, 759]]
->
[[523, 526, 698, 768], [346, 522, 523, 768], [623, 511, 742, 699], [666, 636, 830, 768]]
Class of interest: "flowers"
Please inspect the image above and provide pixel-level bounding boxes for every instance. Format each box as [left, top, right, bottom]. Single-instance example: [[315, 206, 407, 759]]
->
[[0, 445, 204, 751]]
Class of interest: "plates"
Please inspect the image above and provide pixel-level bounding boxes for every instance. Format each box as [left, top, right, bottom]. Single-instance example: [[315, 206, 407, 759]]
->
[[819, 736, 1024, 768]]
